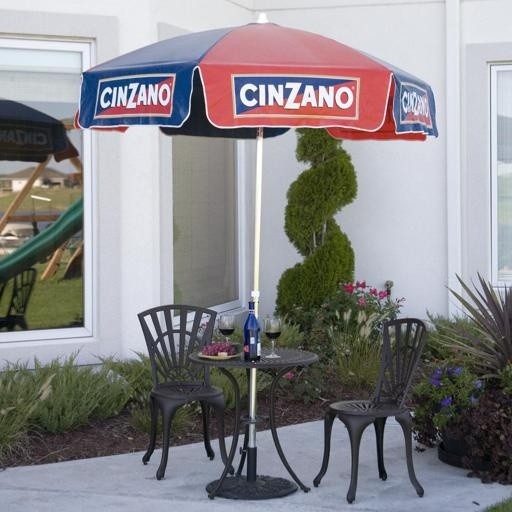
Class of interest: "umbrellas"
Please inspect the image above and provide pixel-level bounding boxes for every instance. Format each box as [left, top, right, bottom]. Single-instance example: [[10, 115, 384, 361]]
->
[[75, 12, 438, 500], [0, 98, 79, 163]]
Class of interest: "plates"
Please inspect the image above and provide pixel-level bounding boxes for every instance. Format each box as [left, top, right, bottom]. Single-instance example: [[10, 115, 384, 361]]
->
[[198, 353, 242, 361]]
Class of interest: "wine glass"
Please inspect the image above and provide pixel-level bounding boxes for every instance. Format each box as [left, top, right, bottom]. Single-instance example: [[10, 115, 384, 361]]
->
[[264, 319, 282, 357], [220, 315, 235, 341]]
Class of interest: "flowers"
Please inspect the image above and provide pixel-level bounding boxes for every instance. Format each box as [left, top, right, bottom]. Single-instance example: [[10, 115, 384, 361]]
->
[[409, 366, 486, 453]]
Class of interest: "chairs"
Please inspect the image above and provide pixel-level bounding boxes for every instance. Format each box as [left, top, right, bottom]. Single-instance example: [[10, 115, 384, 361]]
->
[[137, 304, 234, 480], [313, 318, 427, 503], [0, 268, 37, 331]]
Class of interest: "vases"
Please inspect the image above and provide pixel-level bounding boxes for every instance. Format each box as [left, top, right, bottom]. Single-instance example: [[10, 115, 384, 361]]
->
[[438, 441, 491, 471]]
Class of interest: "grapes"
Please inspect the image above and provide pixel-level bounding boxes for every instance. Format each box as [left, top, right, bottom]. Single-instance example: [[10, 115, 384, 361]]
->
[[202, 342, 238, 356]]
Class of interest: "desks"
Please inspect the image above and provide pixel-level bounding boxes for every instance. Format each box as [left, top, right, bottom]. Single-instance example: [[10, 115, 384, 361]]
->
[[188, 345, 318, 500]]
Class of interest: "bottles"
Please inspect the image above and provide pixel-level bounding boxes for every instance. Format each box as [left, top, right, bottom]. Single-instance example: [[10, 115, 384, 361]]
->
[[242, 301, 261, 362]]
